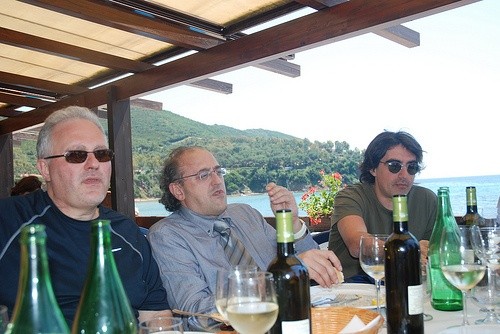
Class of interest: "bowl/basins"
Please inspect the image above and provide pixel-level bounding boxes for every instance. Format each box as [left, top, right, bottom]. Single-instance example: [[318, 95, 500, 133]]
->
[[187, 306, 239, 334]]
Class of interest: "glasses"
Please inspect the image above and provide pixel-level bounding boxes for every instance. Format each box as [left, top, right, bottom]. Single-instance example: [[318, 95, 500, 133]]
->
[[44, 149, 115, 164], [172, 167, 227, 183], [379, 159, 419, 175]]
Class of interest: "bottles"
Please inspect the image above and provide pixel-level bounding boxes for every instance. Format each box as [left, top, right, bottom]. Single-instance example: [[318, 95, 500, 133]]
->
[[459, 186, 488, 287], [382, 195, 425, 334], [71, 220, 138, 334], [4, 225, 71, 334], [266, 209, 311, 334], [425, 186, 464, 311]]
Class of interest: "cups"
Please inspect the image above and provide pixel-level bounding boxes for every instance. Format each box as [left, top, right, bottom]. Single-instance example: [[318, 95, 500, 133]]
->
[[213, 263, 279, 334], [138, 316, 183, 334], [0, 305, 9, 334]]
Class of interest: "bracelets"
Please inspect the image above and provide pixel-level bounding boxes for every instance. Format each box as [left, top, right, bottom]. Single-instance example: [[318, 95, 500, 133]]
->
[[294, 219, 306, 239]]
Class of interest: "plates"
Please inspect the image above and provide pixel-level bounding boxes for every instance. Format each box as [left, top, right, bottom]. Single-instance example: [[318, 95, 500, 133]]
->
[[309, 282, 387, 311]]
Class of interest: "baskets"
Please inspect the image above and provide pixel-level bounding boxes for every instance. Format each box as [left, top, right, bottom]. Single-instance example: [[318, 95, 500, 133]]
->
[[310, 307, 384, 334]]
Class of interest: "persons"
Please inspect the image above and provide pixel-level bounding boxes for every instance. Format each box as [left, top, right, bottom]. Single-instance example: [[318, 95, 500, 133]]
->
[[146, 146, 343, 332], [11, 176, 42, 196], [0, 106, 173, 334], [328, 132, 438, 279]]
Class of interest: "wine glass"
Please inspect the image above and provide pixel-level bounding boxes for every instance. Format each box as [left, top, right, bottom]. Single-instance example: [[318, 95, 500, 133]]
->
[[420, 256, 432, 321], [358, 233, 389, 328], [440, 225, 486, 334], [470, 225, 500, 328]]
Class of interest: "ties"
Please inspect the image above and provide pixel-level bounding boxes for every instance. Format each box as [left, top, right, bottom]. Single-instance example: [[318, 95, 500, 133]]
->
[[213, 219, 263, 278]]
